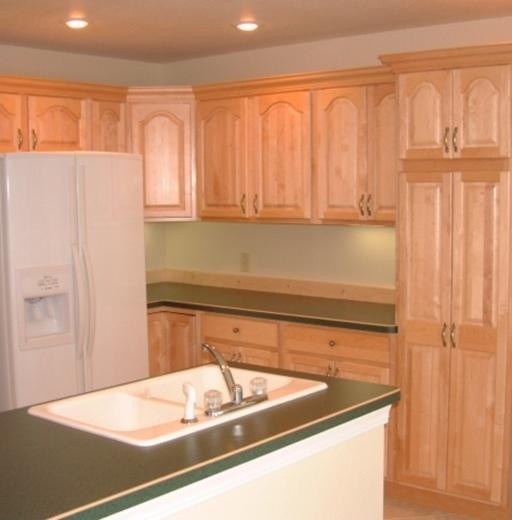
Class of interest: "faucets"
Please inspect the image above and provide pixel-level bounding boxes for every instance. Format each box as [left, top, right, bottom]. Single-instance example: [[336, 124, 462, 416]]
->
[[200, 343, 242, 406]]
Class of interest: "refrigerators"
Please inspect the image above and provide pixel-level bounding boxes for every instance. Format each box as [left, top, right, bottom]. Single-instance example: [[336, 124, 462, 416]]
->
[[0, 152, 149, 411]]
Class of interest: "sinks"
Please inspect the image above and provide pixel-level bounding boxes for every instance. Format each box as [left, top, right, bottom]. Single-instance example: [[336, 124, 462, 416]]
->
[[26, 389, 207, 448], [125, 361, 328, 415]]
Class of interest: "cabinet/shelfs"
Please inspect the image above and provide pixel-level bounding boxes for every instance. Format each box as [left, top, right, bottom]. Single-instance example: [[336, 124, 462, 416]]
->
[[198, 311, 394, 394], [0, 94, 195, 223], [391, 63, 512, 518], [197, 83, 397, 223]]
